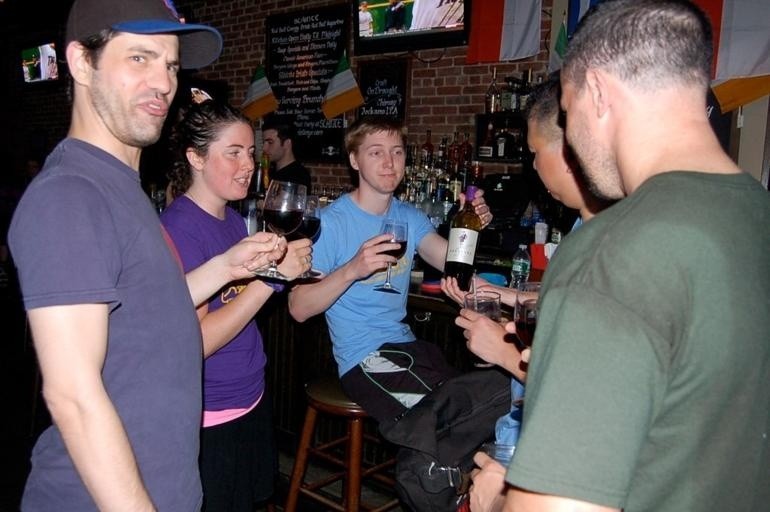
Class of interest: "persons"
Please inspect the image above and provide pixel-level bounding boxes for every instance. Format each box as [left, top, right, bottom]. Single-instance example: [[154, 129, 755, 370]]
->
[[20, 58, 32, 83], [384, 0, 406, 34], [284, 106, 493, 432], [431, 71, 617, 470], [8, 1, 288, 512], [258, 116, 314, 196], [408, 0, 464, 32], [36, 41, 60, 81], [30, 53, 40, 82], [358, 0, 374, 37], [155, 86, 315, 512], [467, 0, 770, 512]]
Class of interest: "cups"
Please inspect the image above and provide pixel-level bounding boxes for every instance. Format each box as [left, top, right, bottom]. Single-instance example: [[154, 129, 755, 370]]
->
[[483, 443, 515, 466], [463, 290, 501, 369]]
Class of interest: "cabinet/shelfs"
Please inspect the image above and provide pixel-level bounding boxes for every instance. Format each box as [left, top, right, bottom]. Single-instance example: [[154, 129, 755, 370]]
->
[[454, 108, 568, 263]]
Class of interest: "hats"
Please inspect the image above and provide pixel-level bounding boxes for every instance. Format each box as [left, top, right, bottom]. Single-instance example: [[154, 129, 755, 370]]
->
[[65, 0, 223, 70]]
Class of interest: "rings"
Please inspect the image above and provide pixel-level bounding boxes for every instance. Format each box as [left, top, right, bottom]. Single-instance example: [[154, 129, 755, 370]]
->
[[304, 255, 312, 264]]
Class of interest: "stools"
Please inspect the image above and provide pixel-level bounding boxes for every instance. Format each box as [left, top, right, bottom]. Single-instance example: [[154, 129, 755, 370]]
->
[[283, 375, 404, 512]]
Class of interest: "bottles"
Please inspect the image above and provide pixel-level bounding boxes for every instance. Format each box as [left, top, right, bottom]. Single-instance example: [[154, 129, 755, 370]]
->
[[443, 185, 484, 292], [243, 162, 266, 236], [508, 243, 532, 289], [484, 65, 500, 112], [500, 68, 543, 113], [399, 130, 478, 185], [478, 119, 522, 164], [399, 185, 442, 215], [444, 186, 461, 221]]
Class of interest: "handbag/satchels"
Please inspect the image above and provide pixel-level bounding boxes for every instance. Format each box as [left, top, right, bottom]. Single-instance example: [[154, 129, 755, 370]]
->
[[378, 364, 511, 511]]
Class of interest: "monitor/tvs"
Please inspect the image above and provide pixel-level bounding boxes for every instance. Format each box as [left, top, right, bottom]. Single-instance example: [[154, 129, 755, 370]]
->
[[352, 0, 471, 56]]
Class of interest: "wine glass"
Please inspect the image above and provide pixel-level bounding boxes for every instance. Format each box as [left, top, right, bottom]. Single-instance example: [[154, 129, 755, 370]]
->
[[512, 279, 545, 409], [372, 218, 410, 296], [251, 178, 310, 280], [297, 195, 322, 277]]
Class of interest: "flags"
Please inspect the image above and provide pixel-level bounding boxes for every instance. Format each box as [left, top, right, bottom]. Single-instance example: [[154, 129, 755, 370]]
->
[[319, 53, 365, 121], [467, 0, 611, 74], [237, 58, 280, 122], [695, 0, 770, 115]]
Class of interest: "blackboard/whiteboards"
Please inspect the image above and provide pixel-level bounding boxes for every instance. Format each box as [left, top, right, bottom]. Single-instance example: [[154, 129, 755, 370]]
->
[[350, 55, 414, 134], [261, 9, 354, 166]]
